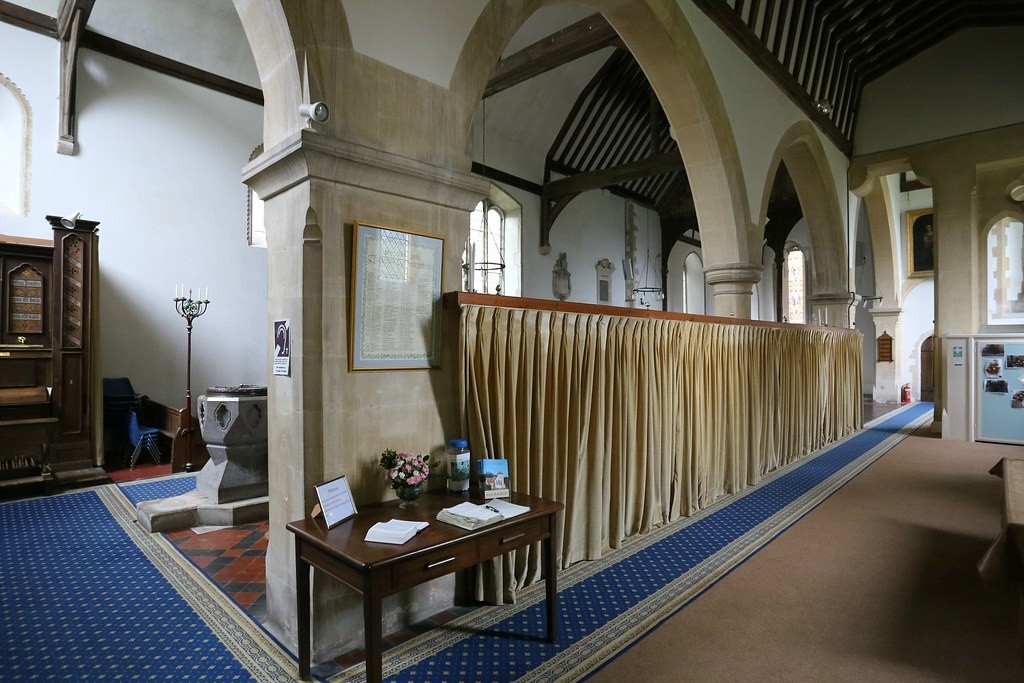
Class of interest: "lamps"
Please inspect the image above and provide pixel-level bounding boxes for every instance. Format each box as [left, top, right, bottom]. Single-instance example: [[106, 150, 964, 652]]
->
[[61, 211, 82, 229], [299, 101, 331, 124]]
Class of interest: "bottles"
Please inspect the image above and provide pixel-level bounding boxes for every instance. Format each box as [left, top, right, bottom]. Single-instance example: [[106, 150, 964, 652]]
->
[[447, 439, 470, 491]]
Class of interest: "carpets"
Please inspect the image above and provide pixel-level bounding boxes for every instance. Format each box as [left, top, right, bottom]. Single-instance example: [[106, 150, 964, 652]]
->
[[0, 401, 934, 683]]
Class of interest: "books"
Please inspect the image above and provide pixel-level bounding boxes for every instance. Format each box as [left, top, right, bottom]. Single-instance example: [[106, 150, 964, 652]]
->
[[436, 498, 531, 530], [476, 458, 510, 499], [364, 518, 429, 545], [0, 455, 39, 471]]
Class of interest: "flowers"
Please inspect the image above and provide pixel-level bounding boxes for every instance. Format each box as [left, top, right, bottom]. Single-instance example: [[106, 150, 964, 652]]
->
[[379, 447, 453, 501]]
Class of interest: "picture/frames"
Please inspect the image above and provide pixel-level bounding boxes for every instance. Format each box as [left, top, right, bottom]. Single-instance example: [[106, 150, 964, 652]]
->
[[905, 207, 936, 278], [310, 475, 358, 530]]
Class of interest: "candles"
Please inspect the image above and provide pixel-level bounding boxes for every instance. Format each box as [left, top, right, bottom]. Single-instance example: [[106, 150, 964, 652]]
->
[[176, 284, 178, 298], [205, 287, 208, 300], [198, 287, 201, 301], [182, 284, 184, 297]]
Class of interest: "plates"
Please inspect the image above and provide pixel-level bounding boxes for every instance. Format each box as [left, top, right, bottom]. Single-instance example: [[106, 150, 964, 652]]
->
[[985, 358, 1002, 377]]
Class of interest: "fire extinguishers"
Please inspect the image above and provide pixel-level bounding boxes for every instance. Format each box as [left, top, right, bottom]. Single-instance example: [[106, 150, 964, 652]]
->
[[901, 383, 911, 403]]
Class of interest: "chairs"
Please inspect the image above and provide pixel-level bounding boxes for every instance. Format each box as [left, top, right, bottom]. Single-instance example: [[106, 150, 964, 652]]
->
[[103, 378, 161, 471]]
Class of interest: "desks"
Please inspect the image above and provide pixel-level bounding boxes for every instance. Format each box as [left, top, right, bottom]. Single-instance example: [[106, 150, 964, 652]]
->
[[978, 456, 1024, 574], [286, 486, 564, 682]]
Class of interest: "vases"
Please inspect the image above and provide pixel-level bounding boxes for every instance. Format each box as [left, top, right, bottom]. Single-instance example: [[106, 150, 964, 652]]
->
[[396, 486, 424, 510]]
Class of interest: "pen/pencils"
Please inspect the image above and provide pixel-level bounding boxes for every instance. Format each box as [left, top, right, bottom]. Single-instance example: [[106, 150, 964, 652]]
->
[[486, 505, 503, 516]]
